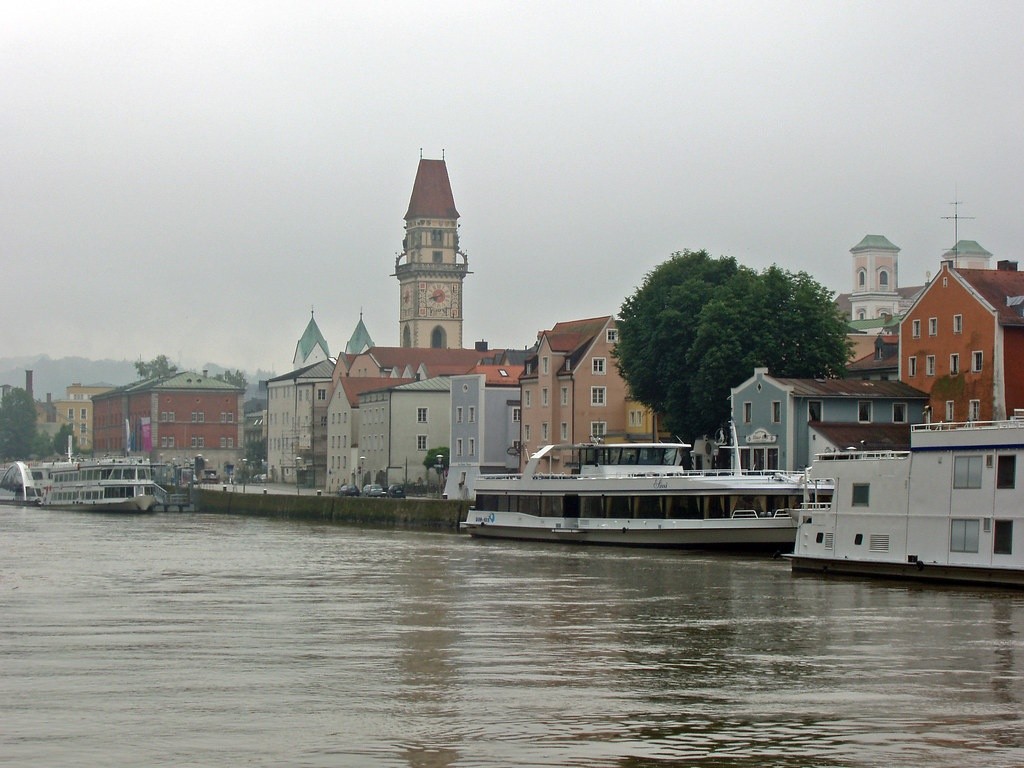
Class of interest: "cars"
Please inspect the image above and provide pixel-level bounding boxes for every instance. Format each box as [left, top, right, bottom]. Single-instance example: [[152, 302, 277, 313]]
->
[[387, 485, 405, 497], [193, 476, 198, 483], [253, 473, 266, 483]]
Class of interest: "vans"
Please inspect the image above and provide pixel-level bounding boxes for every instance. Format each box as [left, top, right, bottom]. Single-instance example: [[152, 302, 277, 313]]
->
[[367, 484, 383, 497]]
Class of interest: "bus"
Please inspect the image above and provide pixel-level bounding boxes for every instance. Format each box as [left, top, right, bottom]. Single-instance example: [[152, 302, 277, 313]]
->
[[200, 469, 216, 483]]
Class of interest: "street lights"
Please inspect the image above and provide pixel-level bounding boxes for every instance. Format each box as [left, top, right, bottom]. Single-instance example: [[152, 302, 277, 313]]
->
[[360, 457, 366, 496], [296, 456, 302, 495], [160, 454, 163, 485], [242, 458, 247, 493], [436, 455, 443, 500]]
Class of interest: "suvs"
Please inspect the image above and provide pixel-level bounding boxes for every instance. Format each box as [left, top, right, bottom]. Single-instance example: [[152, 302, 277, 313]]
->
[[338, 485, 359, 496]]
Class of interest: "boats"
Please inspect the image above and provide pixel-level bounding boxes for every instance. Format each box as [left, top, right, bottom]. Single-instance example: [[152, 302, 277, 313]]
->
[[37, 455, 157, 513], [780, 418, 1024, 591], [458, 441, 798, 547]]
